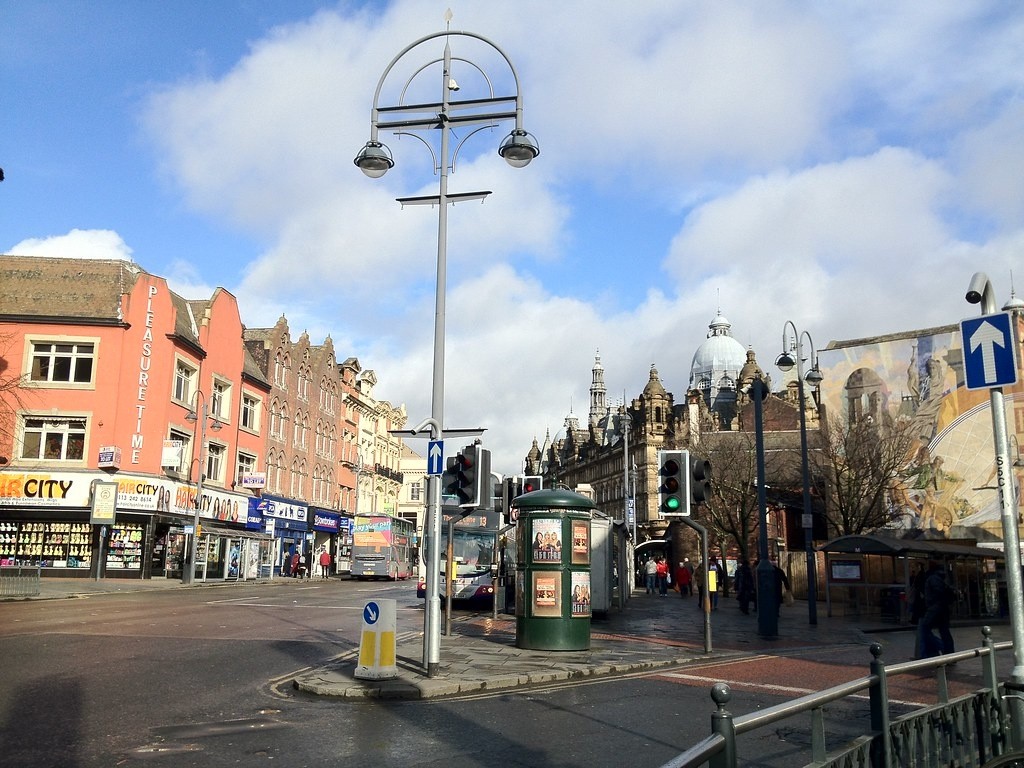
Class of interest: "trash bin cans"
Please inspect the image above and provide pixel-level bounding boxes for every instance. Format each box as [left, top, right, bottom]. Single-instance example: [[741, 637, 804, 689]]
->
[[728, 576, 735, 594]]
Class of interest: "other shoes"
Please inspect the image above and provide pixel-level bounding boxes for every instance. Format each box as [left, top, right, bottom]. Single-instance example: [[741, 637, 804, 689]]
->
[[665, 594, 667, 596], [647, 591, 649, 594], [743, 611, 750, 615], [698, 603, 717, 611], [660, 594, 662, 596], [690, 592, 693, 596], [910, 656, 920, 660], [684, 592, 688, 594], [740, 609, 743, 611], [652, 591, 656, 594]]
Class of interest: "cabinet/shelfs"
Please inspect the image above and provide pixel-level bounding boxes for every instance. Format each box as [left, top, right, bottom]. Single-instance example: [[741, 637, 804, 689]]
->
[[0, 520, 219, 570]]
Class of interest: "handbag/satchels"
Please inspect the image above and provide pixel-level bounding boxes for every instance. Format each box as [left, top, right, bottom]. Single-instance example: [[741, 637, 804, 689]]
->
[[675, 583, 679, 592], [908, 575, 917, 604], [666, 572, 671, 583], [785, 590, 794, 607], [749, 593, 754, 608]]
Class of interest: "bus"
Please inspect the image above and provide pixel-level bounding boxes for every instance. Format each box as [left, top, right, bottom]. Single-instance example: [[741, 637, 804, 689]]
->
[[350, 512, 417, 580], [418, 506, 501, 612]]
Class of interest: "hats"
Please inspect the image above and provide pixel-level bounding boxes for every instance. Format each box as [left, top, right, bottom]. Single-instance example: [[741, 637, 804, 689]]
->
[[710, 555, 716, 563]]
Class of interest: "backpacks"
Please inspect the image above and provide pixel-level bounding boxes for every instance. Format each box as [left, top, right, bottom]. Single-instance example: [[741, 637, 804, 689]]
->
[[931, 575, 953, 605]]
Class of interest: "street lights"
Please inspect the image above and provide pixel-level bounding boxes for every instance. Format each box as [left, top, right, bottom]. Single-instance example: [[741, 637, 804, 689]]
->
[[620, 388, 634, 528], [774, 321, 826, 624], [355, 35, 539, 678], [184, 391, 224, 582]]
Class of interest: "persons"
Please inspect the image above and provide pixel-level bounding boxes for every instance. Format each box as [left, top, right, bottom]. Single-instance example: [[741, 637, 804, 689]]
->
[[230, 542, 240, 574], [674, 555, 723, 611], [212, 497, 239, 522], [282, 550, 307, 579], [886, 563, 957, 667], [320, 549, 330, 579], [900, 359, 965, 530], [571, 585, 590, 612], [157, 485, 170, 512], [734, 560, 790, 618], [636, 555, 672, 598], [533, 531, 561, 559]]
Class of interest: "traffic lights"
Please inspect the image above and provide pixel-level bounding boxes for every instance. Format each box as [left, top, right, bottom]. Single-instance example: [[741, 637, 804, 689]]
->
[[691, 454, 712, 505], [659, 449, 690, 516], [460, 448, 478, 507], [494, 476, 513, 524], [522, 476, 543, 495], [447, 456, 461, 494]]
